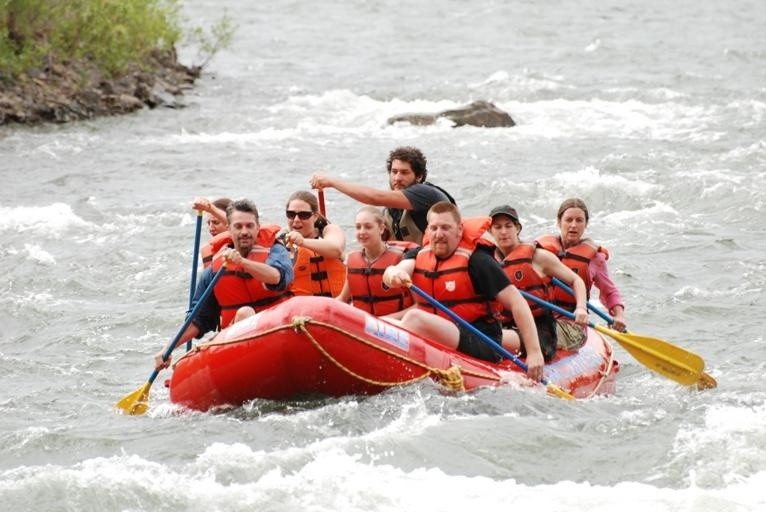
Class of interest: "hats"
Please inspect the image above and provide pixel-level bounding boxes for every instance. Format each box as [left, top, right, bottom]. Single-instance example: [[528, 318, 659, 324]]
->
[[488, 205, 518, 223]]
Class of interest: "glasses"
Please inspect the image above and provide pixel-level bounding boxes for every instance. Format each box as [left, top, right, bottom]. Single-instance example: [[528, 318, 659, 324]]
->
[[286, 211, 312, 220]]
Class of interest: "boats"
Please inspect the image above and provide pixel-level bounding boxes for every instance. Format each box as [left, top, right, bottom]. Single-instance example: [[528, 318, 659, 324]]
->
[[168, 296, 620, 413]]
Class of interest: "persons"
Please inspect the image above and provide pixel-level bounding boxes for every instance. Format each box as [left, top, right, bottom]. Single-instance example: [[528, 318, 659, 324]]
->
[[276, 190, 350, 303], [153, 198, 294, 370], [334, 207, 415, 320], [535, 198, 627, 353], [376, 204, 545, 383], [308, 146, 458, 253], [487, 206, 590, 361], [190, 198, 235, 270], [575, 306, 588, 311]]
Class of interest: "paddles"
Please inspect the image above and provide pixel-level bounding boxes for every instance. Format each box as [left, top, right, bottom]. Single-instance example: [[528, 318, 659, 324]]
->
[[114, 261, 228, 415], [406, 280, 578, 401], [518, 289, 704, 387], [551, 276, 717, 390]]
